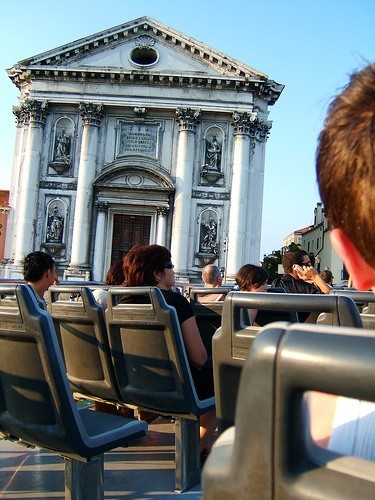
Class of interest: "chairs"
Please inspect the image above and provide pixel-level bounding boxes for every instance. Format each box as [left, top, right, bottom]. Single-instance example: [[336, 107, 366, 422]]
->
[[0, 275, 375, 500]]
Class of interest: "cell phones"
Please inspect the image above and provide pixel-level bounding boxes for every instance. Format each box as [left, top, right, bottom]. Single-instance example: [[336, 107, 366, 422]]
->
[[294, 265, 303, 274]]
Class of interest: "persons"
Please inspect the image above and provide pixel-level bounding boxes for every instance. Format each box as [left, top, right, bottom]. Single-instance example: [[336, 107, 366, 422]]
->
[[234, 264, 268, 327], [197, 264, 226, 302], [320, 270, 334, 286], [202, 217, 216, 249], [205, 135, 222, 170], [23, 252, 57, 309], [118, 243, 219, 401], [90, 259, 131, 309], [54, 127, 70, 161], [208, 62, 375, 462], [48, 207, 63, 240], [270, 249, 332, 324]]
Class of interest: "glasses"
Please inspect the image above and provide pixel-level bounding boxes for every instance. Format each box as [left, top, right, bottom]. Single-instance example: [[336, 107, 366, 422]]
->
[[299, 261, 311, 267], [159, 261, 172, 270]]
